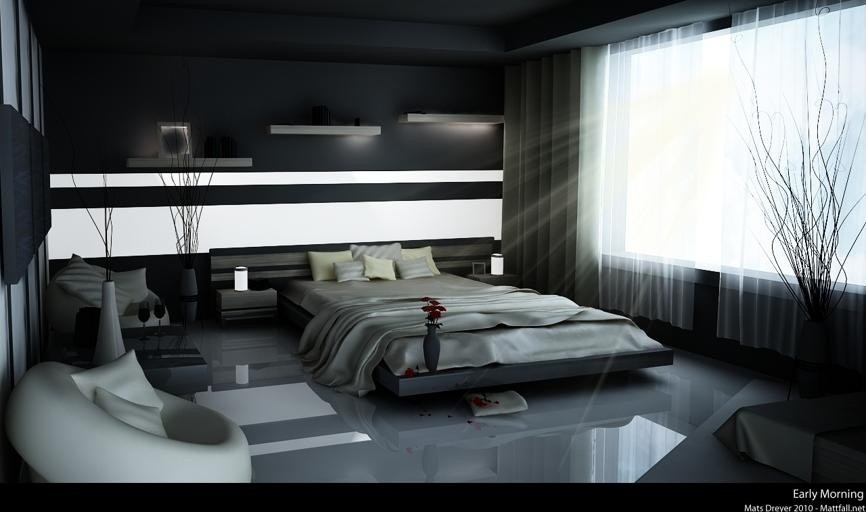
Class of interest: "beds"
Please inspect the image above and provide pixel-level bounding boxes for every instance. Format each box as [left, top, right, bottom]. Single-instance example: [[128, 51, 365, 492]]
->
[[274, 268, 677, 399]]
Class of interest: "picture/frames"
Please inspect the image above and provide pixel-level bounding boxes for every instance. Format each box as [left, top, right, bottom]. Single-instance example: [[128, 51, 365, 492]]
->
[[155, 120, 193, 158]]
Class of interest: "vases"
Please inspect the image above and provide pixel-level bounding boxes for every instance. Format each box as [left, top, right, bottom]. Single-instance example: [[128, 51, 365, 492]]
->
[[93, 279, 128, 366], [802, 322, 825, 365], [424, 325, 442, 374], [183, 269, 199, 324]]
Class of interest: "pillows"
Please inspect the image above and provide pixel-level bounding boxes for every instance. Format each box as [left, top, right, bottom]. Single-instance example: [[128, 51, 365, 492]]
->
[[466, 390, 529, 417], [306, 242, 443, 283], [71, 348, 171, 439], [53, 254, 150, 318]]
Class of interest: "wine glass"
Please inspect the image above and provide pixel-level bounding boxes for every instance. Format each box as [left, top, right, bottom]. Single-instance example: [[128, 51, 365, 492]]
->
[[138, 300, 151, 342], [153, 297, 168, 337]]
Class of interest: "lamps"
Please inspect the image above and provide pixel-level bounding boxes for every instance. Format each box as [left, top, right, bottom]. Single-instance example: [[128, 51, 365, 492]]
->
[[491, 253, 504, 276], [233, 266, 251, 293]]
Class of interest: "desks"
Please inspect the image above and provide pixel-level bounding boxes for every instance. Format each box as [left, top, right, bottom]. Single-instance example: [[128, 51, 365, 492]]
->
[[734, 399, 865, 484]]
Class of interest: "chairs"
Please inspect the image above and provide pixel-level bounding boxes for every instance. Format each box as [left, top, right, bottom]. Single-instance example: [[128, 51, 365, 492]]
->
[[3, 357, 254, 484], [47, 261, 172, 353]]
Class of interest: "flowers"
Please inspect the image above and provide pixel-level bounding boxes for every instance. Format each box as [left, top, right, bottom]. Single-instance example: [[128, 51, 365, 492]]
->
[[473, 397, 488, 407], [418, 297, 446, 328], [404, 364, 420, 379]]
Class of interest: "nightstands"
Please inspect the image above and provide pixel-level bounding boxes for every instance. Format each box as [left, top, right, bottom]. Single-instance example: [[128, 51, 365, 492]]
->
[[215, 287, 278, 328], [469, 272, 522, 287]]
